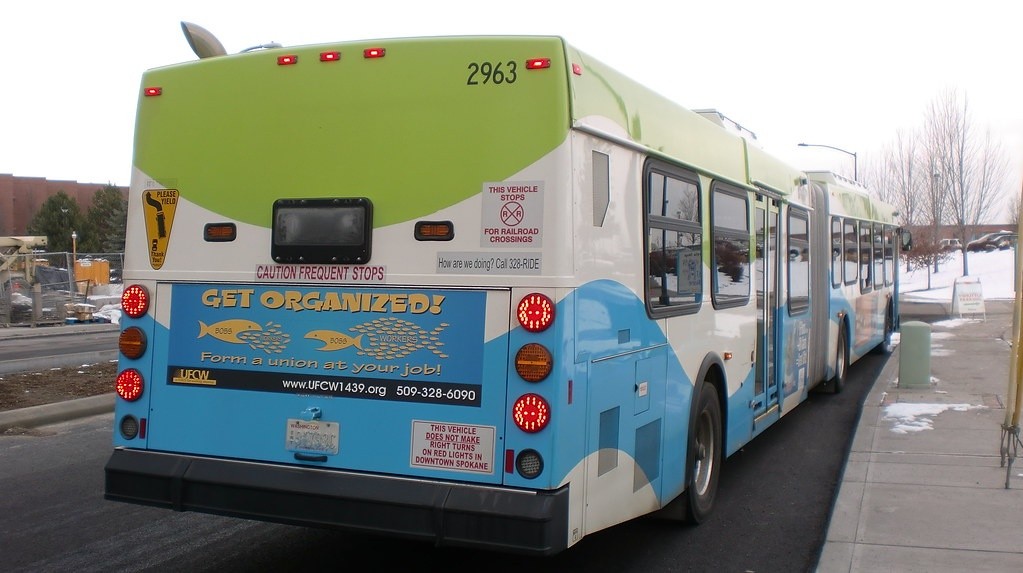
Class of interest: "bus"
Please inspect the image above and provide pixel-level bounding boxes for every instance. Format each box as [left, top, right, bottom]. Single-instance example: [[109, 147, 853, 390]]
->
[[99, 20, 914, 558]]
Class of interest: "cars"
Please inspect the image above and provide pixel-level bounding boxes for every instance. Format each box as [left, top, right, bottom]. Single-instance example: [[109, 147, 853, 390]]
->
[[938, 239, 962, 251], [965, 232, 1017, 252]]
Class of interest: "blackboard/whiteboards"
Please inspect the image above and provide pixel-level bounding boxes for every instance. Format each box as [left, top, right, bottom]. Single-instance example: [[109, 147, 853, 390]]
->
[[951, 276, 985, 314]]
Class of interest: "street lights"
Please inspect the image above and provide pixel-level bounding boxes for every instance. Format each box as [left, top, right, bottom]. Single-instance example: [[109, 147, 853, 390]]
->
[[797, 143, 857, 180]]
